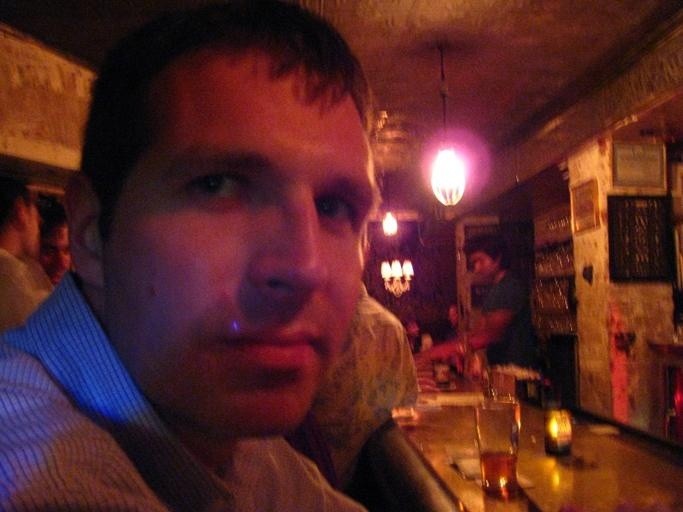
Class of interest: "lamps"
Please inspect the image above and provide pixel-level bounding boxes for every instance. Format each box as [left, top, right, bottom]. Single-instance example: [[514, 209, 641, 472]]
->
[[379, 166, 415, 298], [430, 43, 467, 206]]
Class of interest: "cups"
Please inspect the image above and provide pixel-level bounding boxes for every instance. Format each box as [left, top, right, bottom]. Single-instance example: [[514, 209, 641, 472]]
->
[[473, 401, 521, 499], [543, 409, 572, 456]]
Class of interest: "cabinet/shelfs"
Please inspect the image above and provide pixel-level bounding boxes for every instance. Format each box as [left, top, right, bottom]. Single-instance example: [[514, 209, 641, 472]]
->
[[455, 214, 502, 331]]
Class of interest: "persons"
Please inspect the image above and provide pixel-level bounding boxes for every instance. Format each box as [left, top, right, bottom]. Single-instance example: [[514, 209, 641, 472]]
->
[[0, 1, 371, 512], [442, 304, 458, 339], [0, 174, 56, 331], [402, 320, 432, 354], [30, 191, 71, 288], [423, 233, 545, 407], [283, 271, 421, 492]]
